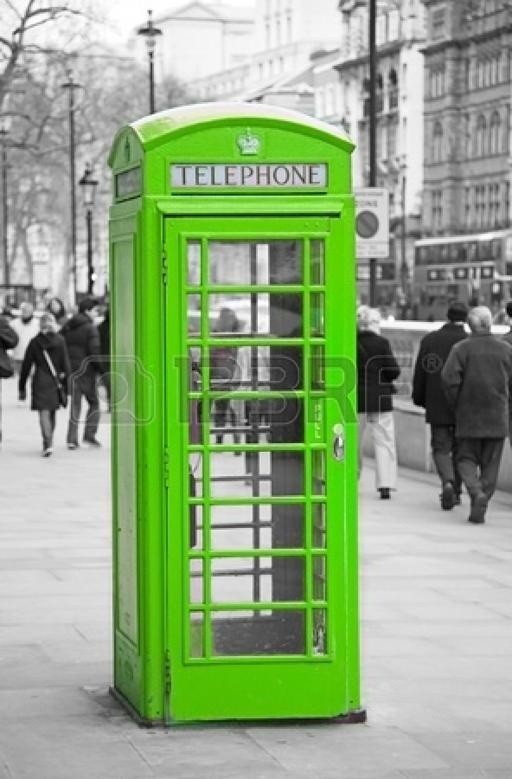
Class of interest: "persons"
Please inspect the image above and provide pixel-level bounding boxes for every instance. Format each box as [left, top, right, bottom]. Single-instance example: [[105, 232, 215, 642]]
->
[[440, 306, 512, 523], [0, 297, 240, 457], [500, 302, 512, 344], [357, 305, 400, 499], [412, 303, 470, 510]]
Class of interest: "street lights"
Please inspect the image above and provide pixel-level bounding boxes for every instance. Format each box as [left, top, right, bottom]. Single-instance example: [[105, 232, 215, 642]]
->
[[137, 4, 162, 118], [76, 162, 103, 325], [59, 71, 87, 328]]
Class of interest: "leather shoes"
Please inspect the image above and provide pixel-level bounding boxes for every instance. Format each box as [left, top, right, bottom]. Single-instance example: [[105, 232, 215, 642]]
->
[[439, 481, 492, 525]]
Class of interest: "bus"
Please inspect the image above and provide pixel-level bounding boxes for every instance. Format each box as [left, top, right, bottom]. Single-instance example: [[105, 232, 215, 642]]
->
[[412, 223, 511, 333]]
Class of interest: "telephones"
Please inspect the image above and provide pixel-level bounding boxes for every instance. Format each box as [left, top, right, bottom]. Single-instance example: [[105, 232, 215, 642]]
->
[[189, 361, 209, 450]]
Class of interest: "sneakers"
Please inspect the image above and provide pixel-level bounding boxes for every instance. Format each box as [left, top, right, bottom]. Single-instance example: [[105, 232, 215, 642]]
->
[[42, 436, 98, 458]]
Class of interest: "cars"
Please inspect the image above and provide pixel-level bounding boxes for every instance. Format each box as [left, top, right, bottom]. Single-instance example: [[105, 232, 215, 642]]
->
[[184, 288, 270, 335]]
